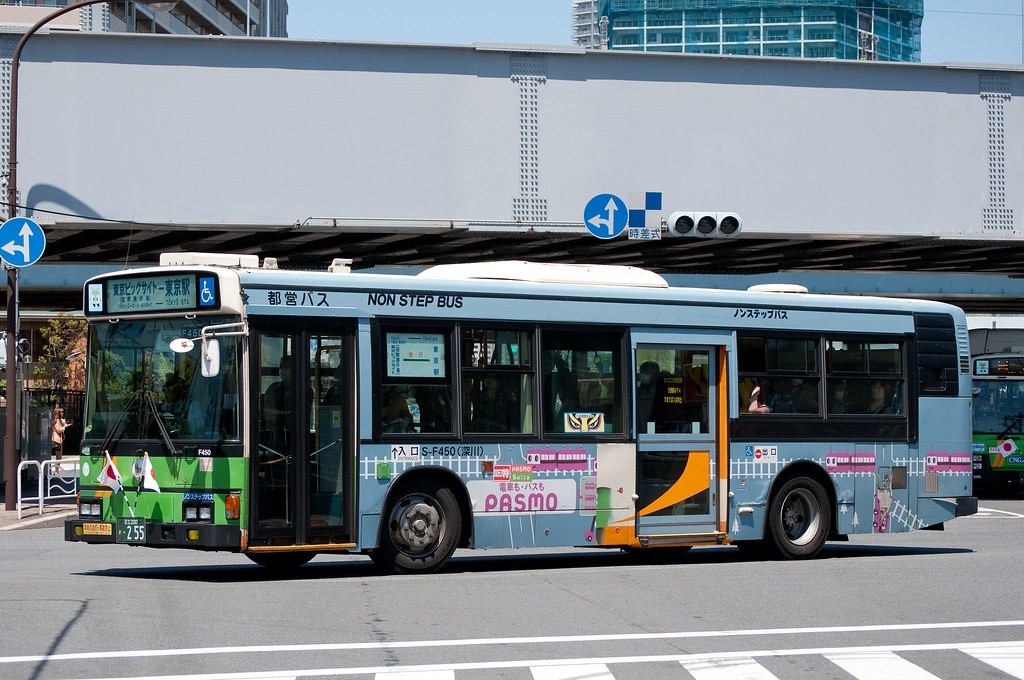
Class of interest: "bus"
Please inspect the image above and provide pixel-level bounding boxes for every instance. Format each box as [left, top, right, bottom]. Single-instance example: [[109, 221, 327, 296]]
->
[[64, 251, 1024, 575]]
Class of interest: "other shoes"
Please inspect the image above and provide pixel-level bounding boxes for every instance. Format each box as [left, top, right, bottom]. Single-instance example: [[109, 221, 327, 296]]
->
[[54, 472, 58, 476]]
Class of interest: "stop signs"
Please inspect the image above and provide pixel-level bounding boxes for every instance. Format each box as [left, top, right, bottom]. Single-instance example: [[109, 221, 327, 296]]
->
[[754, 449, 761, 459]]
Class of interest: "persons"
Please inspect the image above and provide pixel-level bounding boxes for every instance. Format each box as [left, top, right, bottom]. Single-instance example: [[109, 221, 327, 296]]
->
[[264, 356, 314, 432], [382, 361, 894, 433], [978, 394, 995, 416], [153, 373, 192, 419], [50, 407, 72, 475]]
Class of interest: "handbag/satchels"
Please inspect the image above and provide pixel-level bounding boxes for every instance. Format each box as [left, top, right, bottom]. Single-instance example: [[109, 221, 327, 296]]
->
[[51, 441, 61, 451]]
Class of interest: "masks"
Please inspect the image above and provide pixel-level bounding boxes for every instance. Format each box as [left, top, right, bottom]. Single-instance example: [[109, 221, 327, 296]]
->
[[279, 368, 292, 381]]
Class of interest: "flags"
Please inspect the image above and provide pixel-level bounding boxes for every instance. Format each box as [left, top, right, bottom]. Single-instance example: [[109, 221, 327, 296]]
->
[[97, 454, 123, 495], [998, 438, 1017, 457], [141, 455, 161, 494]]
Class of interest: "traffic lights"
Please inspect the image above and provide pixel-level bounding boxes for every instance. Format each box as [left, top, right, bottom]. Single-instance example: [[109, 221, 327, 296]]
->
[[667, 211, 743, 239]]
